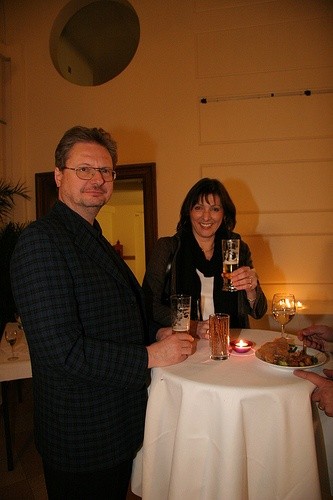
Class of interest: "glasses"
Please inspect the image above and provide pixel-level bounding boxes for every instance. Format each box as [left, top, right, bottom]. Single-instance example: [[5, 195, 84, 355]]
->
[[65, 165, 117, 183]]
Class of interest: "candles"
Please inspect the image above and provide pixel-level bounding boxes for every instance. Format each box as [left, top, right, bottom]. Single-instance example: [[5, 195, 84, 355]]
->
[[235, 340, 248, 350]]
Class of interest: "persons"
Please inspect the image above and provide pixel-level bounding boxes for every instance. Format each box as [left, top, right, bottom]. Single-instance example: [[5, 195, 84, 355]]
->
[[139, 177, 268, 339], [293, 324, 333, 417], [9, 124, 194, 500]]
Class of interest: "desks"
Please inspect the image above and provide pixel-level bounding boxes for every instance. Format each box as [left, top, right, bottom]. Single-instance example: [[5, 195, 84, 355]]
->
[[0, 324, 35, 470], [131, 328, 333, 500], [248, 298, 333, 335]]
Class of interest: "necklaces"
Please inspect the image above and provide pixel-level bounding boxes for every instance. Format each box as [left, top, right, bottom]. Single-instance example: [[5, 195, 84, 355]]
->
[[202, 247, 214, 253]]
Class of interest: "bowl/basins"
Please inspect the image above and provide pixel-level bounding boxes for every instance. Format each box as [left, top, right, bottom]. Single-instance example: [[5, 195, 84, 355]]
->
[[230, 339, 256, 353]]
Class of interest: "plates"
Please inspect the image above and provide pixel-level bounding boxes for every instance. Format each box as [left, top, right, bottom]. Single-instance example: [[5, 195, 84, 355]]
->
[[254, 344, 327, 370]]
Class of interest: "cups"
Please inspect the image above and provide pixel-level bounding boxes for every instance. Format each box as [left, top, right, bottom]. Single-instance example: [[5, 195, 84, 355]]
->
[[170, 294, 192, 335], [208, 313, 230, 361], [221, 239, 240, 291]]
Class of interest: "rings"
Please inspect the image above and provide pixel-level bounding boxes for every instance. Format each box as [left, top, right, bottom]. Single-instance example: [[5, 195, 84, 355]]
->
[[318, 404, 323, 410], [249, 279, 252, 284], [206, 329, 210, 334], [249, 285, 253, 288]]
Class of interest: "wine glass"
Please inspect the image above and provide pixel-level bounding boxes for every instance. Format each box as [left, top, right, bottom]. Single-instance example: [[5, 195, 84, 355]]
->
[[271, 293, 297, 340], [5, 329, 19, 361]]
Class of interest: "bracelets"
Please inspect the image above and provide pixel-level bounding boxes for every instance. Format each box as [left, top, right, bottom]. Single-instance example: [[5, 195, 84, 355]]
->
[[247, 297, 257, 302]]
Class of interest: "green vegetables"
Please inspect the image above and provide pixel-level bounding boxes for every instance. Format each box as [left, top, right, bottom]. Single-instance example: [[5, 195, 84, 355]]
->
[[277, 345, 318, 367]]
